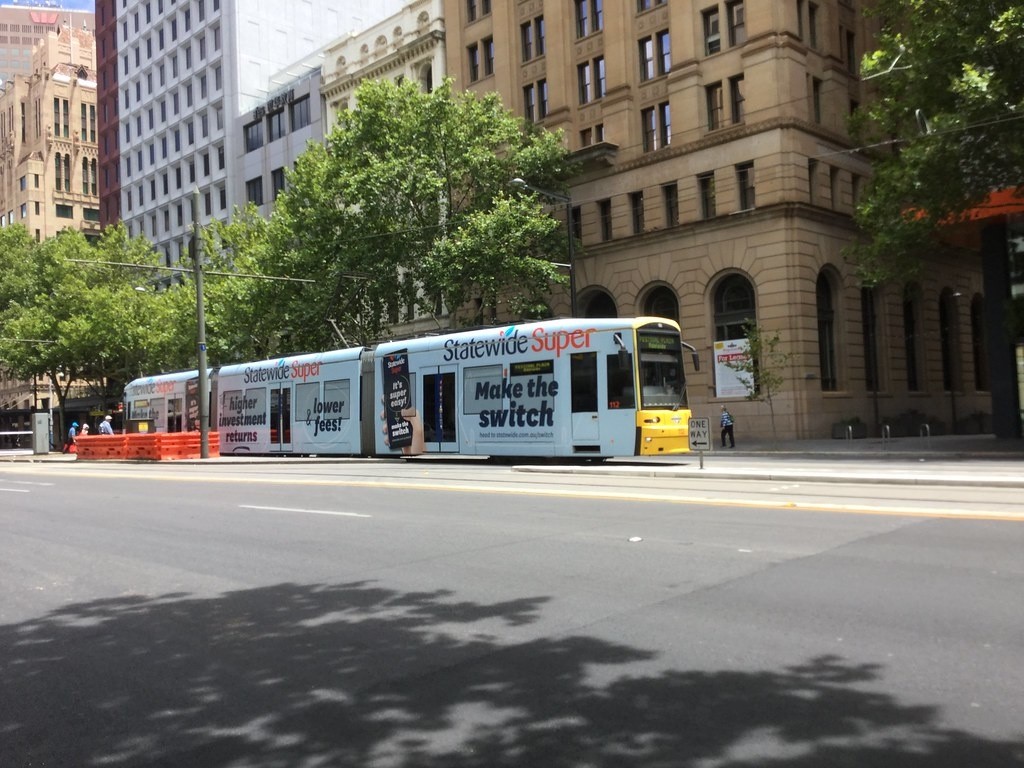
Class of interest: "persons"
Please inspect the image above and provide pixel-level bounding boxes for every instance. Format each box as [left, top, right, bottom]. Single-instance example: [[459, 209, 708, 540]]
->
[[81, 423, 89, 435], [381, 395, 426, 453], [720, 405, 735, 448], [100, 416, 113, 434], [62, 422, 79, 454]]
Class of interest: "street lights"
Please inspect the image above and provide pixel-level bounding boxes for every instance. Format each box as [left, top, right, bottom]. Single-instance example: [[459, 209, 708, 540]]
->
[[508, 177, 577, 318]]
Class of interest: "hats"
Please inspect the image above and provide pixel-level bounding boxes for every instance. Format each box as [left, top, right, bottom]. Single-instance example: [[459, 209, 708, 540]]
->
[[83, 424, 89, 429], [72, 422, 79, 426], [105, 415, 113, 420]]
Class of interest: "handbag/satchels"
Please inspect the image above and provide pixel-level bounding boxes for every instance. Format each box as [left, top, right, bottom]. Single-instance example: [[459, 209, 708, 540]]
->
[[727, 413, 734, 423]]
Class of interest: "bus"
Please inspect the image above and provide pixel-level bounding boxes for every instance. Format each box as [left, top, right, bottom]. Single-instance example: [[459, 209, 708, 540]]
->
[[123, 316, 700, 467]]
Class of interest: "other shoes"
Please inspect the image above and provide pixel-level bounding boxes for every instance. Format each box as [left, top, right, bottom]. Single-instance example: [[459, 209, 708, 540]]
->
[[730, 446, 735, 448], [721, 445, 726, 448]]
